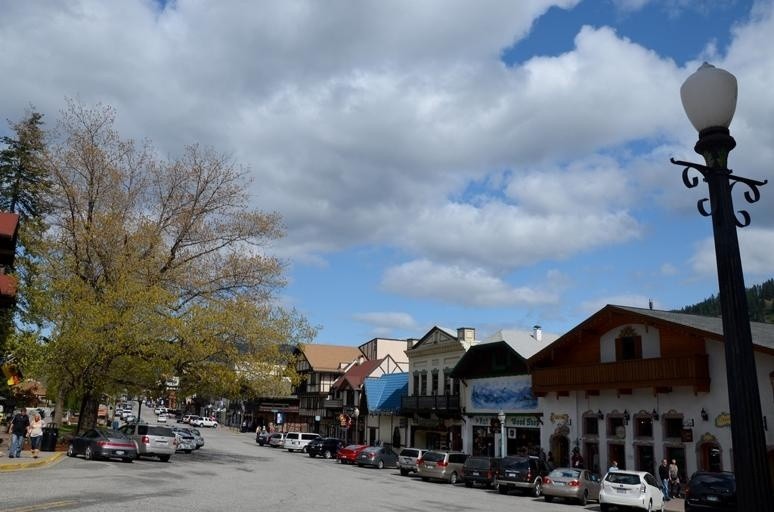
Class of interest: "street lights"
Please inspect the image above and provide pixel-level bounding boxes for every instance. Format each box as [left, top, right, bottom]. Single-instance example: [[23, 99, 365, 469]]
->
[[353, 406, 360, 445], [495, 408, 508, 458], [664, 54, 773, 511]]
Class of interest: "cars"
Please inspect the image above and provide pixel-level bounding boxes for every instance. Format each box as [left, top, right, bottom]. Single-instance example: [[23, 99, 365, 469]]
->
[[395, 444, 599, 508], [114, 393, 221, 463], [252, 430, 397, 472], [64, 427, 138, 465], [597, 468, 667, 512], [682, 469, 736, 512]]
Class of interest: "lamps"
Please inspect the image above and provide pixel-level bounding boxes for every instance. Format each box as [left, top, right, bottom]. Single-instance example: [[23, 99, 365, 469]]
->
[[623, 409, 630, 421], [701, 408, 708, 421], [598, 409, 604, 420], [652, 408, 659, 421]]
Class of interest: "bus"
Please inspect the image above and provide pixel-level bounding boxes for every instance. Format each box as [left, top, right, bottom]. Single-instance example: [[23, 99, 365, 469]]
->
[[0, 394, 71, 427]]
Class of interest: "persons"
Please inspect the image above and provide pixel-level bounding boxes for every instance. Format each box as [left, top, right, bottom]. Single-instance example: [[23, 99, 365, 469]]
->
[[547, 451, 555, 469], [108, 407, 113, 419], [9, 408, 30, 457], [658, 458, 682, 502], [572, 450, 584, 470], [29, 414, 46, 458], [609, 460, 619, 480]]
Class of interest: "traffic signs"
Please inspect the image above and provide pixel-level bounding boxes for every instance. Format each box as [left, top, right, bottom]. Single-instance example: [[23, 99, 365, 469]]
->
[[271, 409, 279, 413]]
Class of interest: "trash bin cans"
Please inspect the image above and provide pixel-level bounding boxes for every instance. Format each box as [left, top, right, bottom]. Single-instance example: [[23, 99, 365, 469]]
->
[[41, 423, 58, 452]]
